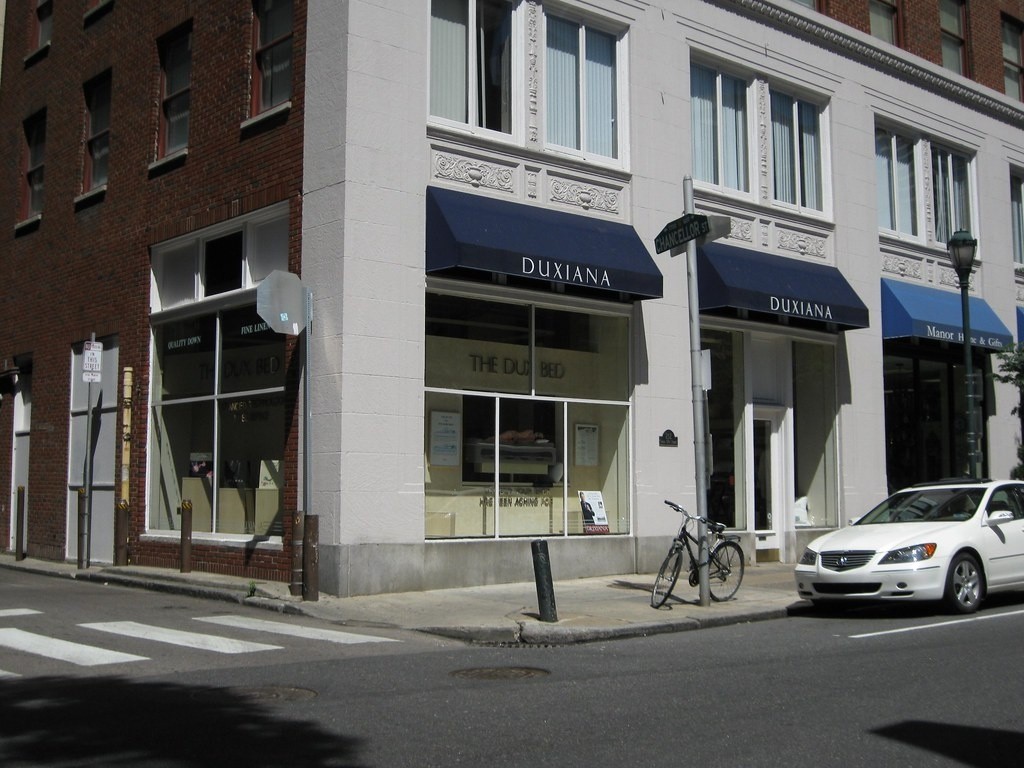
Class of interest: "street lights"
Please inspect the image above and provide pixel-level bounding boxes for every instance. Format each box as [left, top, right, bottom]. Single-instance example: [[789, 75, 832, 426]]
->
[[946, 227, 978, 480]]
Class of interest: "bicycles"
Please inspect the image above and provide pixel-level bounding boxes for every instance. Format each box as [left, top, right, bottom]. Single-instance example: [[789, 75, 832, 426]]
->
[[650, 500, 746, 608]]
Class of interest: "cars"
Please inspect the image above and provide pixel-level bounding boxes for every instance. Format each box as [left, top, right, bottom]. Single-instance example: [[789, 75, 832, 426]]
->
[[793, 480, 1023, 614]]
